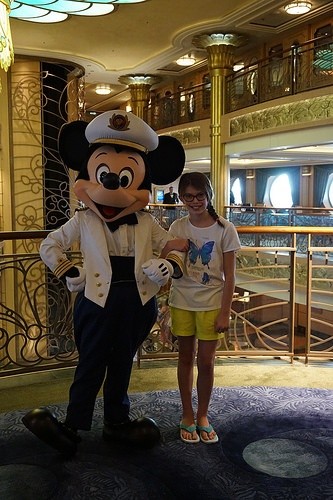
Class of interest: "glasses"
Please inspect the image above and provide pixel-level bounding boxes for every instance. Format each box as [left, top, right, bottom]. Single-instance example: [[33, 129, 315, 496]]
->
[[182, 192, 209, 203]]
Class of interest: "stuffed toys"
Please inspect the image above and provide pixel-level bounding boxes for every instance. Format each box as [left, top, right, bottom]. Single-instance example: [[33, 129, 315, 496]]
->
[[20, 109, 187, 454]]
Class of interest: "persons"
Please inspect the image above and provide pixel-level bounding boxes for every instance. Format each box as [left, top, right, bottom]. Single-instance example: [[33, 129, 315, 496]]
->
[[158, 172, 242, 443], [156, 90, 174, 126], [162, 186, 179, 210]]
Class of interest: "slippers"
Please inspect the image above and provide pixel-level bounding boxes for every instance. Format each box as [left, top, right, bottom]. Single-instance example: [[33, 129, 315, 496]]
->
[[179, 421, 200, 443], [195, 420, 218, 444]]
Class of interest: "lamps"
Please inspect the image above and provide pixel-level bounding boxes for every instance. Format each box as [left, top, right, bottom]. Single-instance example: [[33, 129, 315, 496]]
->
[[285, 2, 311, 15], [96, 84, 112, 94], [176, 53, 196, 66]]
